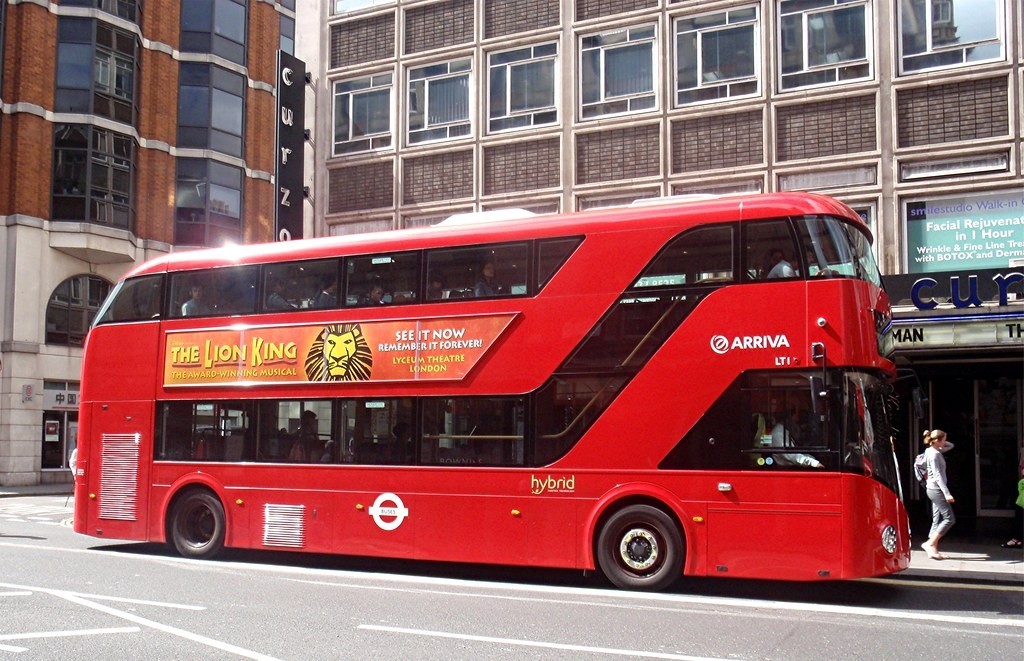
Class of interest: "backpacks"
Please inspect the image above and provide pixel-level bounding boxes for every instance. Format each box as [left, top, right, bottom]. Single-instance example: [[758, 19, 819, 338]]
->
[[914, 453, 929, 481]]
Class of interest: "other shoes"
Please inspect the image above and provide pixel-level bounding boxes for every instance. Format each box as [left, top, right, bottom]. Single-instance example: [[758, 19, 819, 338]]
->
[[921, 542, 950, 560]]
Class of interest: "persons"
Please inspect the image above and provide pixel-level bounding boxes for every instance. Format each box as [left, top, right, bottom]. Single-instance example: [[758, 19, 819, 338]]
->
[[921, 429, 956, 560], [842, 440, 857, 466], [182, 256, 498, 318], [1000, 475, 1024, 549], [767, 242, 816, 278], [390, 396, 452, 467], [745, 392, 827, 468], [318, 439, 339, 464], [279, 408, 316, 463]]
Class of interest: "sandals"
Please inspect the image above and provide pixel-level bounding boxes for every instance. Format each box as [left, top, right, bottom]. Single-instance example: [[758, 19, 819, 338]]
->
[[1000, 539, 1022, 549]]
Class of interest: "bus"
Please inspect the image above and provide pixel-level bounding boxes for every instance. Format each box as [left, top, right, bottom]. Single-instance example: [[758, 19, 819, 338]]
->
[[72, 189, 913, 592]]
